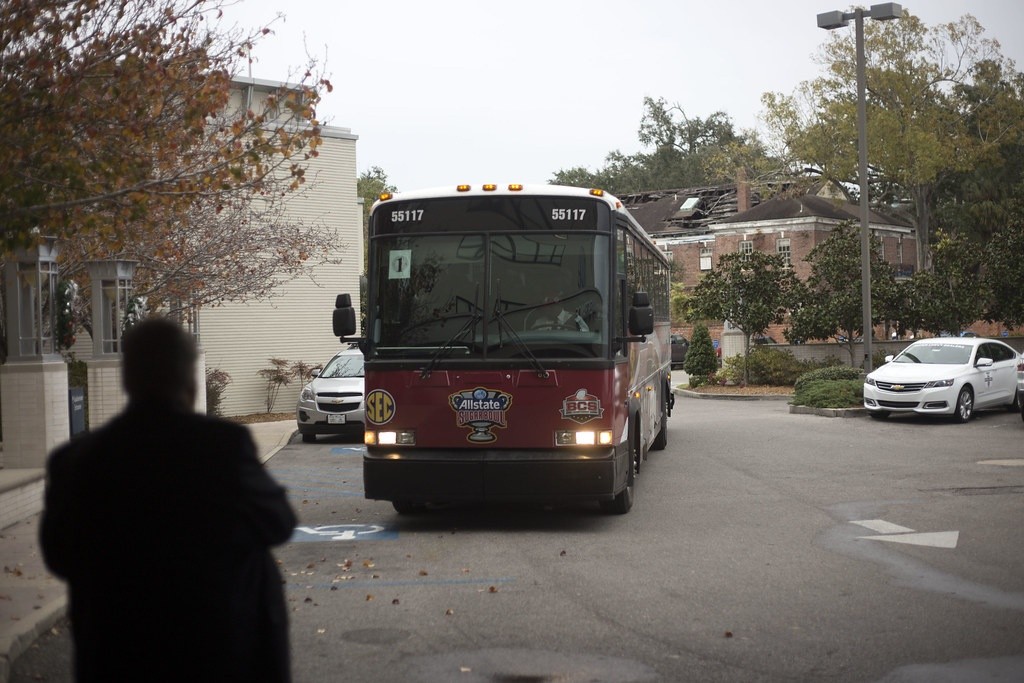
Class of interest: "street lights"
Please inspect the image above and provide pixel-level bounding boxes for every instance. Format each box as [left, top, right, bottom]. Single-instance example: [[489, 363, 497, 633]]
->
[[817, 2, 902, 380]]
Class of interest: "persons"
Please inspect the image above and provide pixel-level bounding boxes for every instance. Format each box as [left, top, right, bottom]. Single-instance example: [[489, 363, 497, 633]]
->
[[530, 289, 589, 332], [37, 315, 299, 683]]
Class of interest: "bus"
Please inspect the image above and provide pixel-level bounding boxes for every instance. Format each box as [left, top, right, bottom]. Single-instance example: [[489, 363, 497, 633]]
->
[[331, 182, 677, 515]]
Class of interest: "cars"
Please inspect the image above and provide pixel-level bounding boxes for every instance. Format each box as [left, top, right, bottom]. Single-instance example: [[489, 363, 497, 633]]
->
[[296, 348, 366, 443], [936, 331, 979, 338], [1017, 352, 1024, 421], [863, 337, 1022, 424], [671, 335, 690, 370]]
